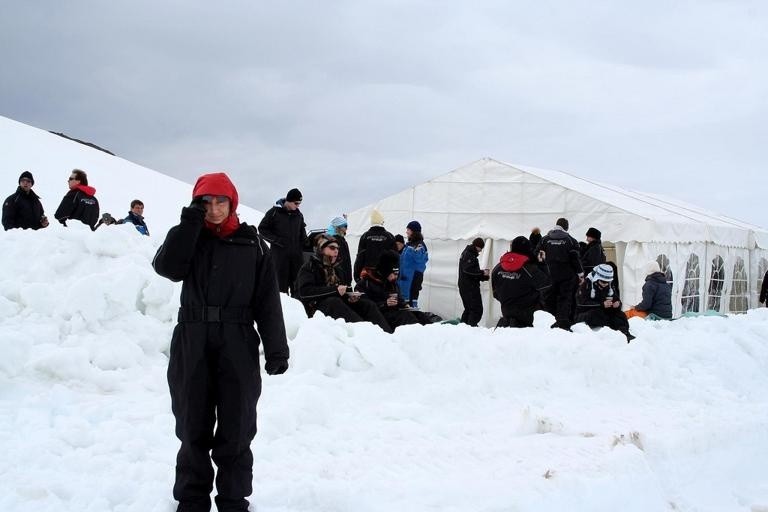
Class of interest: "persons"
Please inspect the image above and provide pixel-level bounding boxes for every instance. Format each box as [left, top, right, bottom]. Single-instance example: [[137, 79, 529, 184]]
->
[[759, 270, 768, 308], [257, 188, 442, 334], [2, 171, 49, 231], [150, 172, 290, 512], [53, 168, 99, 232], [122, 200, 149, 236], [458, 238, 490, 327], [624, 260, 672, 321], [491, 218, 636, 343]]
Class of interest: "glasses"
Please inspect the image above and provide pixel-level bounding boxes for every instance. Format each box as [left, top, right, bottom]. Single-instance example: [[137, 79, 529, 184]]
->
[[294, 202, 301, 206], [69, 177, 76, 181], [326, 244, 339, 251]]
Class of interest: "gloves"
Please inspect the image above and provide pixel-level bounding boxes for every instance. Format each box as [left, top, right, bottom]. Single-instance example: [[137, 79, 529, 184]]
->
[[188, 197, 207, 212], [263, 358, 290, 375]]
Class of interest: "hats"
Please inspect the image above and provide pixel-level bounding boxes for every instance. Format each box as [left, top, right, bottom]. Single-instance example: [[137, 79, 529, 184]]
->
[[18, 170, 34, 186], [472, 237, 485, 249], [588, 262, 614, 299], [394, 233, 405, 244], [555, 217, 570, 231], [331, 216, 348, 228], [315, 233, 339, 251], [376, 249, 401, 277], [405, 221, 421, 232], [286, 188, 303, 202], [586, 227, 602, 239], [529, 227, 543, 244], [369, 206, 384, 226], [644, 260, 660, 275], [509, 235, 531, 253]]
